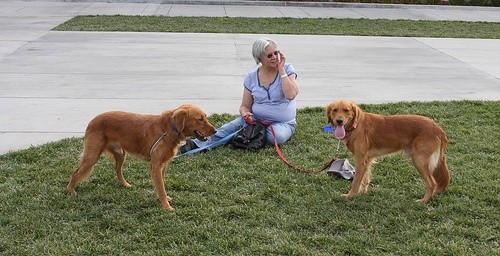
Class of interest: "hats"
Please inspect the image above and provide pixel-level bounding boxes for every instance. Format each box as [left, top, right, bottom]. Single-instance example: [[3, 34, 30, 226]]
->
[[327, 159, 355, 180]]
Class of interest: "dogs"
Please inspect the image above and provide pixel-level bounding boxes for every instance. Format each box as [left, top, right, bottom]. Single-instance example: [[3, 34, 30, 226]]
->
[[326, 99, 450, 204], [68, 104, 217, 211]]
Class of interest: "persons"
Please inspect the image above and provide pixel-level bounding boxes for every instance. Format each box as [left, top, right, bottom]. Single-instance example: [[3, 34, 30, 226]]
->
[[179, 37, 299, 156]]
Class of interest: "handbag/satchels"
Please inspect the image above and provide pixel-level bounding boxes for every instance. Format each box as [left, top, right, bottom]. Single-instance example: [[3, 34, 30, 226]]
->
[[233, 122, 266, 151]]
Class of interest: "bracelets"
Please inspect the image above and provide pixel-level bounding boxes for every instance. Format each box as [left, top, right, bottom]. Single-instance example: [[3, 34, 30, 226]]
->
[[281, 74, 288, 78]]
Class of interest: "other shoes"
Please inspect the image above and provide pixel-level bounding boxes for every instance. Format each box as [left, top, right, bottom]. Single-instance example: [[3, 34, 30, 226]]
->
[[181, 140, 192, 153]]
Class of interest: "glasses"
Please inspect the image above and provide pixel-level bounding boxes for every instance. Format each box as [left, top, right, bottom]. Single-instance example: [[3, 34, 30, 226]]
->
[[264, 51, 279, 58]]
